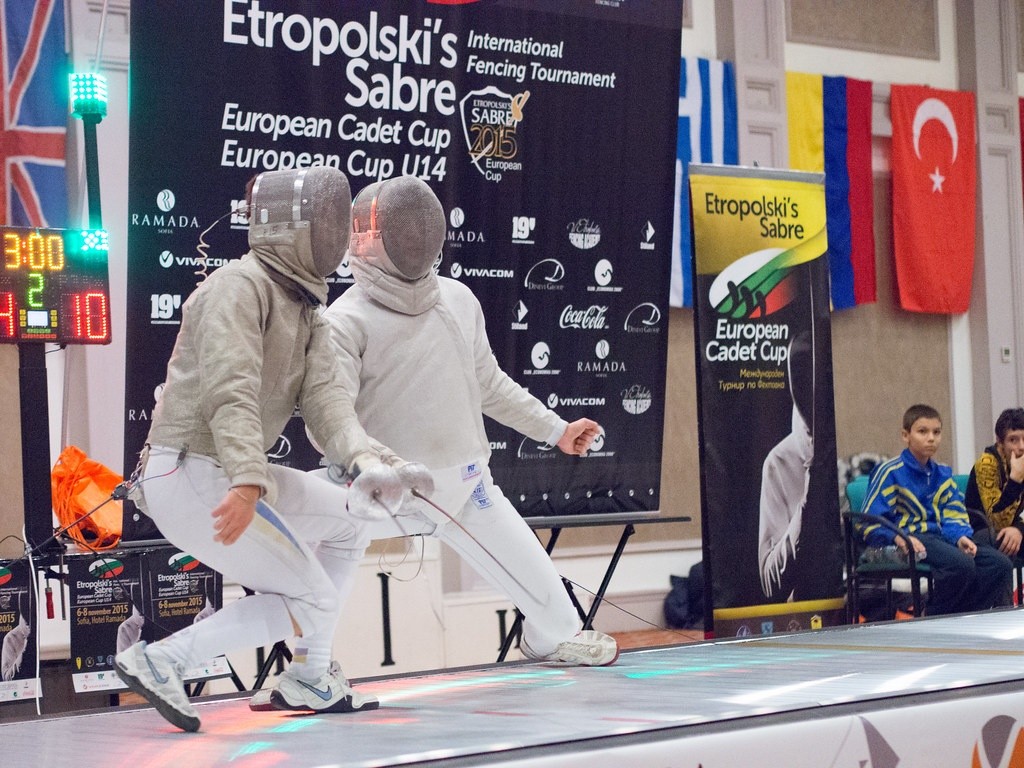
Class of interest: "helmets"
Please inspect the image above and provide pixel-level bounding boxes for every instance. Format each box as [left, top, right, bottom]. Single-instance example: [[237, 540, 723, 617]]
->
[[248, 166, 351, 306], [350, 173, 446, 317]]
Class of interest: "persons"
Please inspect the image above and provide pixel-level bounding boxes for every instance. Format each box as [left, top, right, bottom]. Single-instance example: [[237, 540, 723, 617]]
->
[[758, 329, 843, 603], [249, 175, 621, 712], [109, 166, 386, 732], [193, 574, 213, 624], [966, 409, 1024, 608], [862, 406, 1004, 617], [116, 578, 145, 654], [1, 591, 37, 681]]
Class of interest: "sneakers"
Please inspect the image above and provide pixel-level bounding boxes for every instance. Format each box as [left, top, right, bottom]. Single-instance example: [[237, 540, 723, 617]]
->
[[111, 641, 202, 733], [270, 660, 379, 713], [249, 660, 352, 712], [521, 630, 618, 667]]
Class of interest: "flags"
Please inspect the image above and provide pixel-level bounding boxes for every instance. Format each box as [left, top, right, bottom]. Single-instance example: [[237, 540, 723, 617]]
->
[[0, 0, 70, 231], [889, 84, 977, 315], [784, 72, 878, 311], [668, 58, 740, 308]]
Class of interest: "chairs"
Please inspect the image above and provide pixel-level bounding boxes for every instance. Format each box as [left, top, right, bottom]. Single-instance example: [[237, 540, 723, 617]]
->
[[951, 474, 1024, 606], [842, 474, 933, 626]]
